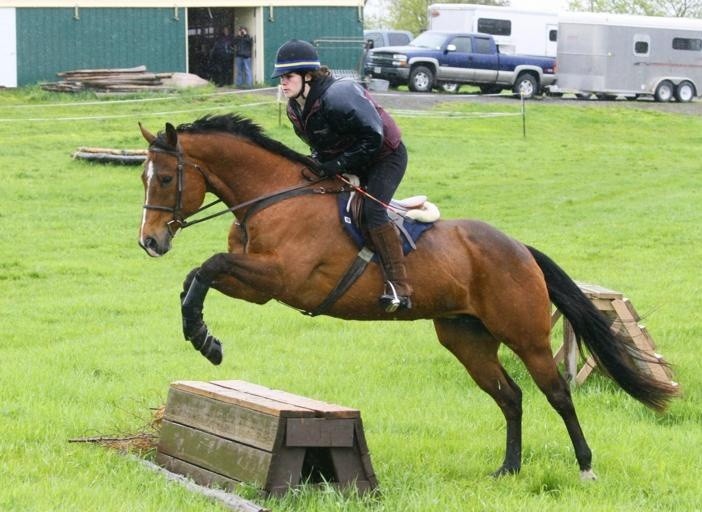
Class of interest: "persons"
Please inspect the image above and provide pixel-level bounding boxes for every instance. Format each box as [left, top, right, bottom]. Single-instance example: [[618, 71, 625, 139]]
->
[[231, 25, 254, 89], [270, 37, 412, 315], [213, 25, 233, 87]]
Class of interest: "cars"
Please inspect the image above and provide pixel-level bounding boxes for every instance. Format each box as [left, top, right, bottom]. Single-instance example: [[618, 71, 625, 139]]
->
[[364, 28, 412, 49]]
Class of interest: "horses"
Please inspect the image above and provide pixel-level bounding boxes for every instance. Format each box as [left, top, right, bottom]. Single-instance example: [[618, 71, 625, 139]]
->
[[133, 112, 678, 483]]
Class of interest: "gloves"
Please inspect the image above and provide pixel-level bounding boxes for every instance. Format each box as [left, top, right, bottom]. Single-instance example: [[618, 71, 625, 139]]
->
[[316, 159, 339, 177]]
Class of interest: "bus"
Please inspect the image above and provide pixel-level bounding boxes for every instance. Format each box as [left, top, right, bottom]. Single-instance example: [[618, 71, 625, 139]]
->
[[428, 3, 702, 102]]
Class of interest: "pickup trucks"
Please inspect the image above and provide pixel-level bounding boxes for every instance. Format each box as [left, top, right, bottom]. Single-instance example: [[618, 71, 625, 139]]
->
[[364, 32, 557, 98]]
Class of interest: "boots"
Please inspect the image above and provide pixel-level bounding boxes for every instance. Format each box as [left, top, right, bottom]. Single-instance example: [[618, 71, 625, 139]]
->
[[369, 221, 412, 311]]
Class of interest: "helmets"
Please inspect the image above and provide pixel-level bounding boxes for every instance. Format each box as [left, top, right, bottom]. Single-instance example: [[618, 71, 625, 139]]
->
[[270, 38, 320, 79]]
